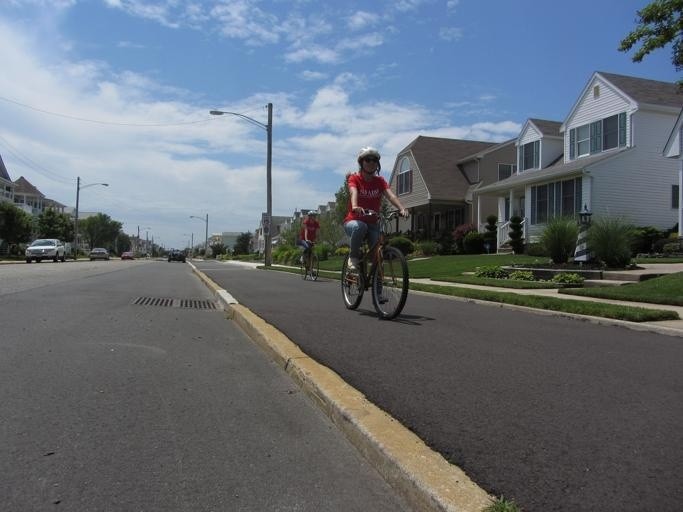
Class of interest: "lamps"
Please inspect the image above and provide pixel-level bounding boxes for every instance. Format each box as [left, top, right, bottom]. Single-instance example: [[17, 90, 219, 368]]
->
[[572, 202, 595, 261]]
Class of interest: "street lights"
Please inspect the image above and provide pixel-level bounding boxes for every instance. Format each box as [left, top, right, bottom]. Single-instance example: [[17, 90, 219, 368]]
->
[[208, 108, 273, 268], [189, 215, 208, 251], [72, 183, 109, 260], [183, 232, 193, 251], [136, 224, 151, 250]]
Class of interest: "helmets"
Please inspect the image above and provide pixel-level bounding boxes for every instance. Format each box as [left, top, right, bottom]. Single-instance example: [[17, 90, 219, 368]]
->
[[308, 211, 318, 216], [358, 147, 380, 162]]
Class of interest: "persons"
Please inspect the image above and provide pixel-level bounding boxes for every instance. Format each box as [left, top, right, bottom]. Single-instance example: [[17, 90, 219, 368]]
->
[[343, 147, 409, 303], [299, 210, 321, 277]]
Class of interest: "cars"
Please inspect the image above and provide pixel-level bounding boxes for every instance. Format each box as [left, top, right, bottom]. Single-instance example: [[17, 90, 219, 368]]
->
[[24, 237, 66, 264], [87, 246, 110, 261], [120, 252, 134, 260], [167, 249, 187, 262]]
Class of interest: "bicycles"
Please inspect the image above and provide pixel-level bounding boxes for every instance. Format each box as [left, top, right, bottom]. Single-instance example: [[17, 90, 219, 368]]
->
[[298, 240, 321, 281], [337, 205, 410, 321]]
